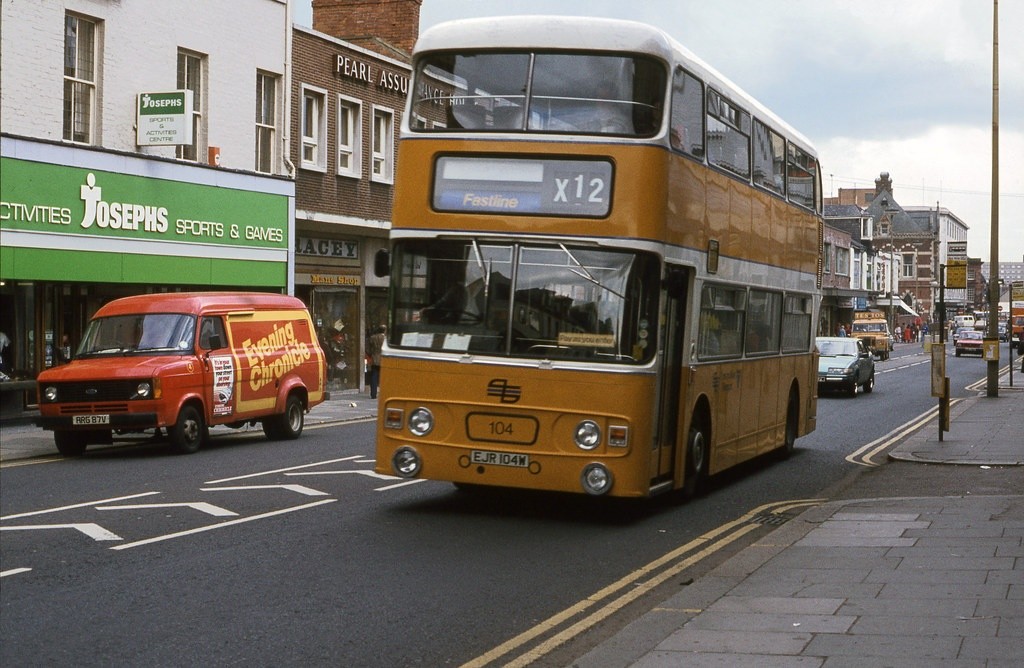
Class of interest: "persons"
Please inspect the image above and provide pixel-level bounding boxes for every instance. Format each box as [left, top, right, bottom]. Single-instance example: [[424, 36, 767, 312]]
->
[[834, 322, 851, 337], [894, 321, 918, 343], [365, 325, 386, 399], [578, 80, 633, 134], [419, 249, 497, 327], [920, 321, 929, 348]]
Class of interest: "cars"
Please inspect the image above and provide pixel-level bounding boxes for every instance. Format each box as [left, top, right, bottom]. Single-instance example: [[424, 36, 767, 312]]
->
[[952, 326, 975, 346], [974, 320, 989, 331], [955, 330, 985, 357], [995, 322, 1010, 342], [813, 335, 876, 397]]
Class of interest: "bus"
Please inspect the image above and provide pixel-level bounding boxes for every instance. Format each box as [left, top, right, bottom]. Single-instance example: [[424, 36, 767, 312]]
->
[[370, 9, 827, 514]]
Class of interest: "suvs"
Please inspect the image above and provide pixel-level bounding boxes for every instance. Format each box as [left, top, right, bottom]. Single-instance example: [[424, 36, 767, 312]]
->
[[850, 316, 891, 361]]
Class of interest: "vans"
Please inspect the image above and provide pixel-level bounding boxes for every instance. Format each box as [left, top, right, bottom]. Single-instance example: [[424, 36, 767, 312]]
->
[[33, 285, 330, 455], [954, 315, 975, 326]]
